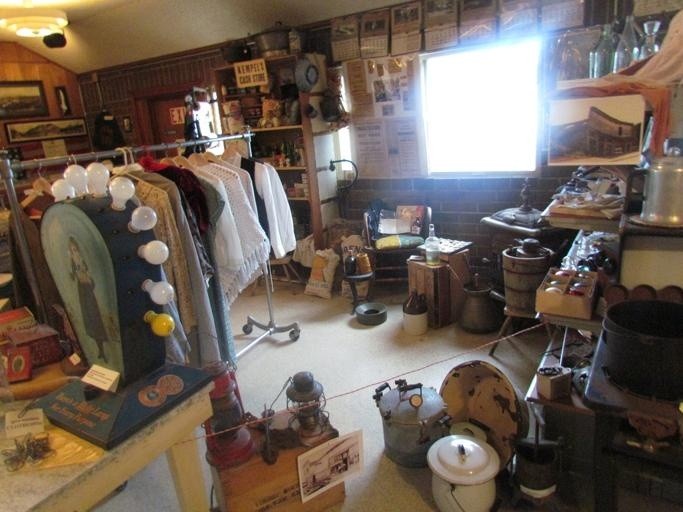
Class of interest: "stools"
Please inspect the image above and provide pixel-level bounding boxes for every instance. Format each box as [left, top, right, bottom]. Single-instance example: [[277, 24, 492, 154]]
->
[[248, 255, 304, 295]]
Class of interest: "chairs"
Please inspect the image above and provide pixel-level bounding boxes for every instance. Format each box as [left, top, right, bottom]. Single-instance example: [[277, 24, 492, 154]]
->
[[362, 195, 433, 302]]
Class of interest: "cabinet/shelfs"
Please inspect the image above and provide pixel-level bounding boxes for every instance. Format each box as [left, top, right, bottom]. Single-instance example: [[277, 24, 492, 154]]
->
[[209, 53, 342, 284]]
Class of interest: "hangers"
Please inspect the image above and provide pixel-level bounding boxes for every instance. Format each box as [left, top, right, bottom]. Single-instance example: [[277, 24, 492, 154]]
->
[[0, 132, 269, 223]]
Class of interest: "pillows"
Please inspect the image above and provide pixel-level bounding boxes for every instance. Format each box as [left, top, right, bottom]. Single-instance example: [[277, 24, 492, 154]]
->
[[374, 234, 424, 251]]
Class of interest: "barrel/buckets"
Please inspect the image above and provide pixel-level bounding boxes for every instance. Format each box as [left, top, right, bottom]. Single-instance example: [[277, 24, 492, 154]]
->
[[402, 290, 427, 332], [502, 245, 556, 312]]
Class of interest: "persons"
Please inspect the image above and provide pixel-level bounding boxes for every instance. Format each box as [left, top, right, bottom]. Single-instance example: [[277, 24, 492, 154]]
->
[[63, 236, 109, 364]]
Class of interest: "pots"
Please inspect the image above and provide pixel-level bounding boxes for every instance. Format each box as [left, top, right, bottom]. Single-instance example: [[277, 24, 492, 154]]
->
[[375, 379, 452, 471]]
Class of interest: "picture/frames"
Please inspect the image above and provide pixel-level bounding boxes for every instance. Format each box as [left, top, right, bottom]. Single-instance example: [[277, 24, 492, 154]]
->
[[0, 80, 88, 158]]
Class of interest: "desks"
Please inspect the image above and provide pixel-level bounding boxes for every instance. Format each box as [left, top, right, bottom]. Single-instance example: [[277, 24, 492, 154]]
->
[[0, 379, 211, 511]]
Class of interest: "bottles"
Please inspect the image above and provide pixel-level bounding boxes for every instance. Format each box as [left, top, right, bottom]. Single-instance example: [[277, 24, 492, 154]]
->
[[412, 216, 422, 236], [424, 223, 440, 265], [344, 249, 358, 276], [401, 288, 429, 336], [588, 14, 640, 79]]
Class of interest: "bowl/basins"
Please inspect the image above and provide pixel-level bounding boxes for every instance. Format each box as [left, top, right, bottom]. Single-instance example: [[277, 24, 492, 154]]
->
[[440, 360, 530, 474]]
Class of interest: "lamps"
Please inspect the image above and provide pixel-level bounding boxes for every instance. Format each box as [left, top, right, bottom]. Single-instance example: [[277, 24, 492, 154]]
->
[[0, 9, 67, 38]]
